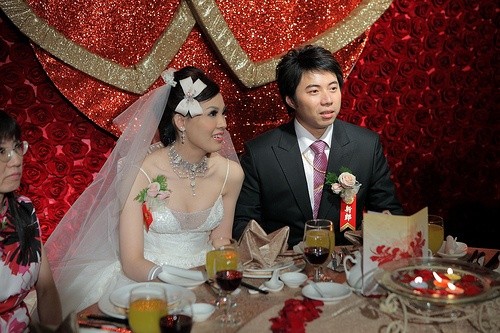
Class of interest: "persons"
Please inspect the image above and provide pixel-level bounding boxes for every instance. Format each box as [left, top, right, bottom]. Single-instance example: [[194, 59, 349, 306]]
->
[[0, 110, 62, 333], [232, 44, 402, 250], [26, 65, 245, 326]]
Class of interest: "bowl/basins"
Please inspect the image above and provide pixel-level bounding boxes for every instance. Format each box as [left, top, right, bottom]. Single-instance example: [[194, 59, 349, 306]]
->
[[279, 272, 308, 288], [376, 257, 500, 305], [190, 303, 216, 322]]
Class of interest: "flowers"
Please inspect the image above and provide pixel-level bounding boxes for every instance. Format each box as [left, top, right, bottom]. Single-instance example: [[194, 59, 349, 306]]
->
[[134, 175, 173, 210], [325, 167, 361, 205]]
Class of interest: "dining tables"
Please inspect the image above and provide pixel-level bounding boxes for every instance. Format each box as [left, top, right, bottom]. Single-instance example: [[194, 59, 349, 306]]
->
[[77, 248, 500, 333]]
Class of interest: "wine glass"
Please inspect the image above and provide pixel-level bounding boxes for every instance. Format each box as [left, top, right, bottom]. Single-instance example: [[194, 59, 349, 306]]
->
[[302, 219, 335, 283], [215, 246, 243, 327], [205, 239, 240, 308]]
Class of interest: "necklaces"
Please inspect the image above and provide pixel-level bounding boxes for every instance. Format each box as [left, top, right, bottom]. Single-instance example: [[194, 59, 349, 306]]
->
[[169, 146, 207, 196], [302, 152, 326, 174]]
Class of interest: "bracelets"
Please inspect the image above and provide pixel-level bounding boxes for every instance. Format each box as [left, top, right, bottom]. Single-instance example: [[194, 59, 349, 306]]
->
[[151, 267, 160, 281]]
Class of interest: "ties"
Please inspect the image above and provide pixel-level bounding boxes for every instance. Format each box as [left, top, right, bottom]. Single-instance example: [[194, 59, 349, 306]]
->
[[309, 139, 328, 225]]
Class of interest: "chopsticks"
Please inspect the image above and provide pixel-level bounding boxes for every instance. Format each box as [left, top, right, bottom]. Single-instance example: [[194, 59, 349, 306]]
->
[[240, 281, 268, 296]]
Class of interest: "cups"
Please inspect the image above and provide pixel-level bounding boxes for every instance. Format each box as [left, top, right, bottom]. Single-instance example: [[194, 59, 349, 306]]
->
[[159, 296, 193, 333], [421, 214, 444, 257], [128, 284, 167, 333]]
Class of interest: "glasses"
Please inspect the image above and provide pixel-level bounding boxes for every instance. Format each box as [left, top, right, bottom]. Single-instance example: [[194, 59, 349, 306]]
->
[[0, 141, 28, 162]]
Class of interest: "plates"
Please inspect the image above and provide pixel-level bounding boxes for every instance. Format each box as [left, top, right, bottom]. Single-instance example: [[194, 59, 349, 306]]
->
[[369, 288, 489, 324], [110, 284, 183, 309], [302, 281, 352, 304], [240, 250, 307, 278], [243, 256, 294, 273], [326, 260, 350, 272], [436, 240, 468, 259], [157, 269, 208, 290], [98, 285, 195, 319]]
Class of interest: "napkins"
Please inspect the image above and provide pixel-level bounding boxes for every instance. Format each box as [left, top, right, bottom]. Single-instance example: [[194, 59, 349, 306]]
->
[[239, 219, 291, 266], [344, 210, 391, 250], [28, 311, 78, 333]]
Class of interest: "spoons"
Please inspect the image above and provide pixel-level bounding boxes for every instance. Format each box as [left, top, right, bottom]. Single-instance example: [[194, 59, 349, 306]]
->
[[265, 268, 284, 293]]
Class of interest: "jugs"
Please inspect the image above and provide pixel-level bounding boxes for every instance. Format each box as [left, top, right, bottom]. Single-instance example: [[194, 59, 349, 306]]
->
[[344, 250, 362, 288]]
